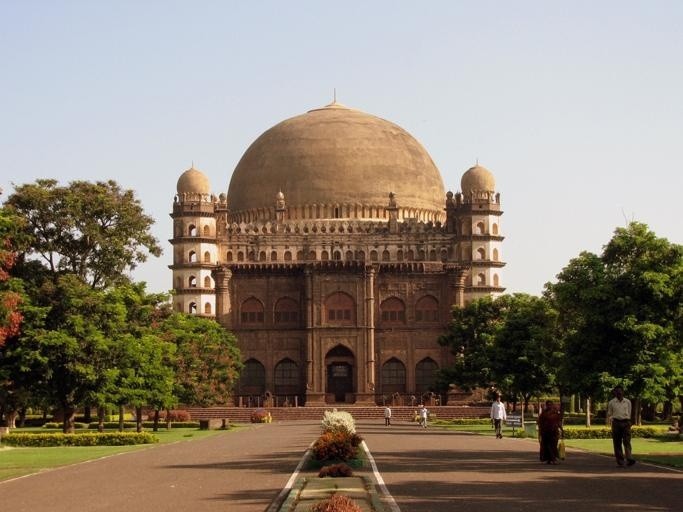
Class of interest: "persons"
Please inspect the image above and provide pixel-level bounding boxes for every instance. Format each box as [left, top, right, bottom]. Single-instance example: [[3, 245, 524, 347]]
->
[[490, 395, 507, 439], [420, 406, 428, 428], [538, 399, 566, 465], [607, 385, 636, 466], [384, 405, 392, 426]]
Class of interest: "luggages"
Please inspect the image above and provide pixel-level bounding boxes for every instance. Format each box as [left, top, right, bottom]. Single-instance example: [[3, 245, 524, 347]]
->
[[559, 432, 566, 459]]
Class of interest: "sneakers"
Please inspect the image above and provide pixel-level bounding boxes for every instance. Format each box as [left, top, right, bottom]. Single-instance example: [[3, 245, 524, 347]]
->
[[496, 434, 503, 439], [627, 458, 635, 467]]
